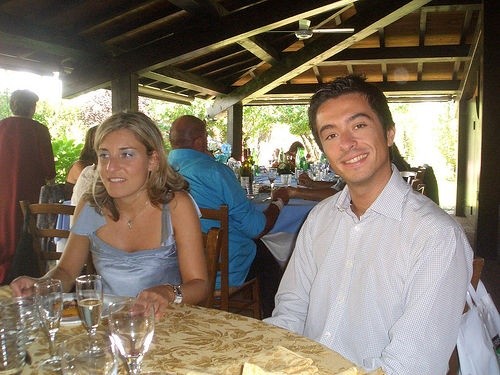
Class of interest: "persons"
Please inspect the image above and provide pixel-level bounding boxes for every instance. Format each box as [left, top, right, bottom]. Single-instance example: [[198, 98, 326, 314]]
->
[[389, 142, 410, 170], [1, 89, 57, 284], [283, 170, 346, 201], [7, 110, 210, 321], [63, 123, 99, 202], [272, 142, 304, 175], [260, 73, 473, 375], [166, 115, 289, 316]]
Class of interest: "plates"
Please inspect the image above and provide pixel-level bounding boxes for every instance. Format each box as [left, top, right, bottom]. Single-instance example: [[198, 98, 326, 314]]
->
[[41, 292, 124, 326]]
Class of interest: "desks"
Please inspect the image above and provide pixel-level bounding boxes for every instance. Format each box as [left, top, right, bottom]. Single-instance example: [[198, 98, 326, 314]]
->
[[247, 197, 321, 266], [0, 288, 386, 375]]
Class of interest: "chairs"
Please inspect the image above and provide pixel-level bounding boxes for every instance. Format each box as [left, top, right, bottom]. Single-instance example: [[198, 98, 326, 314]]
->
[[197, 225, 221, 314], [198, 202, 263, 320], [447, 254, 485, 375], [19, 197, 93, 283], [400, 168, 424, 187]]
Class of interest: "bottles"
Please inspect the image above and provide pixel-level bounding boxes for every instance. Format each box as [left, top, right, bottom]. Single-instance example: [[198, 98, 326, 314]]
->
[[230, 148, 255, 192], [273, 146, 309, 176]]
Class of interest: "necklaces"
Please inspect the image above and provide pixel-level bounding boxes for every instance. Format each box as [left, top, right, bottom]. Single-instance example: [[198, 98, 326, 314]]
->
[[113, 198, 153, 231]]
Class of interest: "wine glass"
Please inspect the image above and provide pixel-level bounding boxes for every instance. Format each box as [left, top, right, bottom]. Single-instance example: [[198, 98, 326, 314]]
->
[[108, 297, 155, 375], [74, 274, 103, 356], [33, 278, 63, 370], [267, 168, 277, 200]]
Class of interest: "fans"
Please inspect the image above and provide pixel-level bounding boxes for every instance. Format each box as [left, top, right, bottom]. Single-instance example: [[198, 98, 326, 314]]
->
[[266, 19, 355, 41]]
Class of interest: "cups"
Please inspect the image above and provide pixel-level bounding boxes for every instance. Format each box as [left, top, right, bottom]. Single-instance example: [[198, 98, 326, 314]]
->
[[246, 183, 259, 199], [280, 174, 292, 186], [15, 296, 40, 345], [59, 331, 119, 375], [0, 298, 26, 375], [295, 163, 340, 185]]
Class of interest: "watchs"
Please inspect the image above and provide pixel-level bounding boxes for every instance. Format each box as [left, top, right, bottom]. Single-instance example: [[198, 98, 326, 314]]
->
[[167, 284, 184, 305]]
